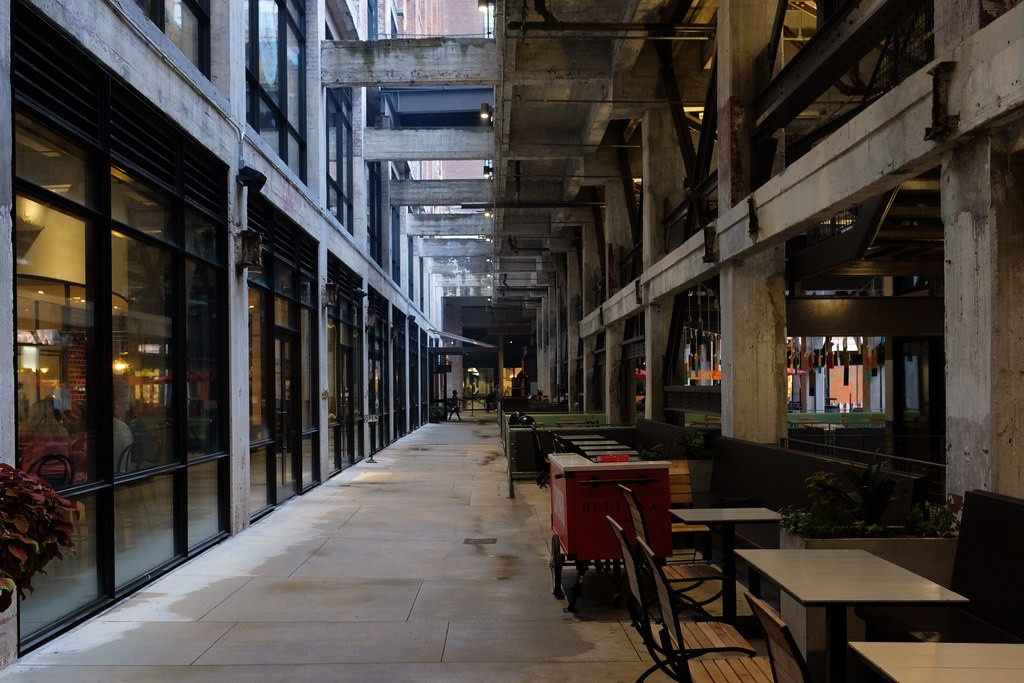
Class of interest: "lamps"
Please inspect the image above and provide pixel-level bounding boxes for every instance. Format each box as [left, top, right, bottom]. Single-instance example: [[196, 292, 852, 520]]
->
[[486, 234, 492, 242], [483, 166, 494, 178], [236, 166, 267, 190], [347, 287, 367, 308], [477, 0, 494, 11], [113, 356, 129, 375], [480, 103, 493, 118], [486, 256, 492, 262], [485, 207, 493, 216]]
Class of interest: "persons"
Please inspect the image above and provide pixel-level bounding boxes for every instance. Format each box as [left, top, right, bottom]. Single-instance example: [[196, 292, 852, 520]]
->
[[448, 390, 460, 420], [16, 381, 153, 492]]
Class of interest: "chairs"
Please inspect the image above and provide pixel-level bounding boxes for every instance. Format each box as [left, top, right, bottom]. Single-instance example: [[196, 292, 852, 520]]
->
[[637, 536, 774, 683], [617, 484, 724, 624], [606, 515, 757, 683], [531, 425, 554, 486], [539, 434, 567, 489], [664, 460, 711, 559], [744, 591, 814, 683], [26, 454, 78, 491], [113, 435, 164, 534]]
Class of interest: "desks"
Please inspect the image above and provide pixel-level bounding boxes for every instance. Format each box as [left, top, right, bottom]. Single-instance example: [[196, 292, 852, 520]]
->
[[571, 440, 618, 445], [559, 434, 604, 441], [591, 457, 638, 463], [584, 450, 639, 457], [849, 640, 1024, 683], [579, 446, 629, 451], [669, 509, 789, 626], [735, 549, 969, 683]]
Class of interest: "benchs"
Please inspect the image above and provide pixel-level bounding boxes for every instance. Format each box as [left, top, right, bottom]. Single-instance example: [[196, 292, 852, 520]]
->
[[498, 401, 605, 452], [853, 489, 1024, 642], [505, 420, 637, 498]]
